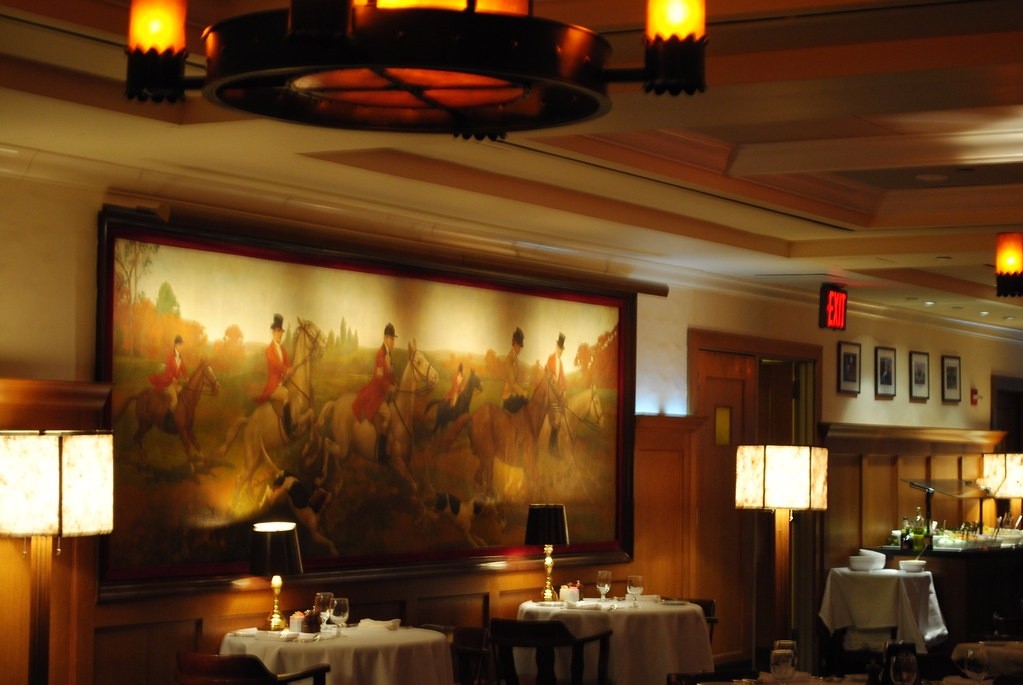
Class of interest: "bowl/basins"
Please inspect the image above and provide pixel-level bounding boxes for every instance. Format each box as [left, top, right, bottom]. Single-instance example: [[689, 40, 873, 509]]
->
[[899, 560, 927, 573], [849, 555, 877, 570], [859, 549, 887, 570]]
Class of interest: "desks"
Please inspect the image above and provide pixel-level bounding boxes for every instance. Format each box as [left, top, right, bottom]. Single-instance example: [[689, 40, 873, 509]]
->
[[867, 536, 1023, 677], [218, 625, 453, 685], [519, 596, 713, 685], [820, 566, 947, 653]]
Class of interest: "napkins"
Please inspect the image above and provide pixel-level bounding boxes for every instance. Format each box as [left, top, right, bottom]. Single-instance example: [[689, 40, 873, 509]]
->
[[358, 619, 401, 632]]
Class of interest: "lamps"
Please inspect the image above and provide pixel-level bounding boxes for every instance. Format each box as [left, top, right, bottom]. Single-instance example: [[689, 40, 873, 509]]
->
[[980, 452, 1023, 527], [0, 426, 113, 685], [254, 521, 296, 577], [733, 445, 828, 639], [524, 502, 569, 602], [125, 0, 711, 144]]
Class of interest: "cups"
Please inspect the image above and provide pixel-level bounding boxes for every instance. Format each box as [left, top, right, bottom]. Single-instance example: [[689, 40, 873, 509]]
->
[[891, 655, 917, 685], [773, 640, 798, 676]]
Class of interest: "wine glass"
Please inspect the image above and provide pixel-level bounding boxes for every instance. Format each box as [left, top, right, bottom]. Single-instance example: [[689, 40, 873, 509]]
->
[[329, 598, 349, 639], [770, 648, 796, 685], [627, 575, 643, 609], [595, 569, 612, 603], [963, 648, 990, 685], [315, 592, 334, 632]]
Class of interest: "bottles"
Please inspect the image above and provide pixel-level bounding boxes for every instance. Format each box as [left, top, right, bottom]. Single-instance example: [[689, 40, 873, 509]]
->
[[880, 642, 922, 685], [901, 516, 912, 550], [912, 507, 925, 553]]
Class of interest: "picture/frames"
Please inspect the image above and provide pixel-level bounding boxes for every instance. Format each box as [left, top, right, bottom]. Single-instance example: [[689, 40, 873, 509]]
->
[[909, 351, 930, 399], [90, 204, 637, 606], [874, 346, 897, 396], [836, 340, 861, 394], [942, 355, 962, 401]]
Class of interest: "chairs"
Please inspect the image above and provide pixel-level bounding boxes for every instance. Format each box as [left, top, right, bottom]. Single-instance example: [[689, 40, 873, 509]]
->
[[491, 617, 613, 685], [175, 649, 332, 685], [663, 596, 719, 685]]
